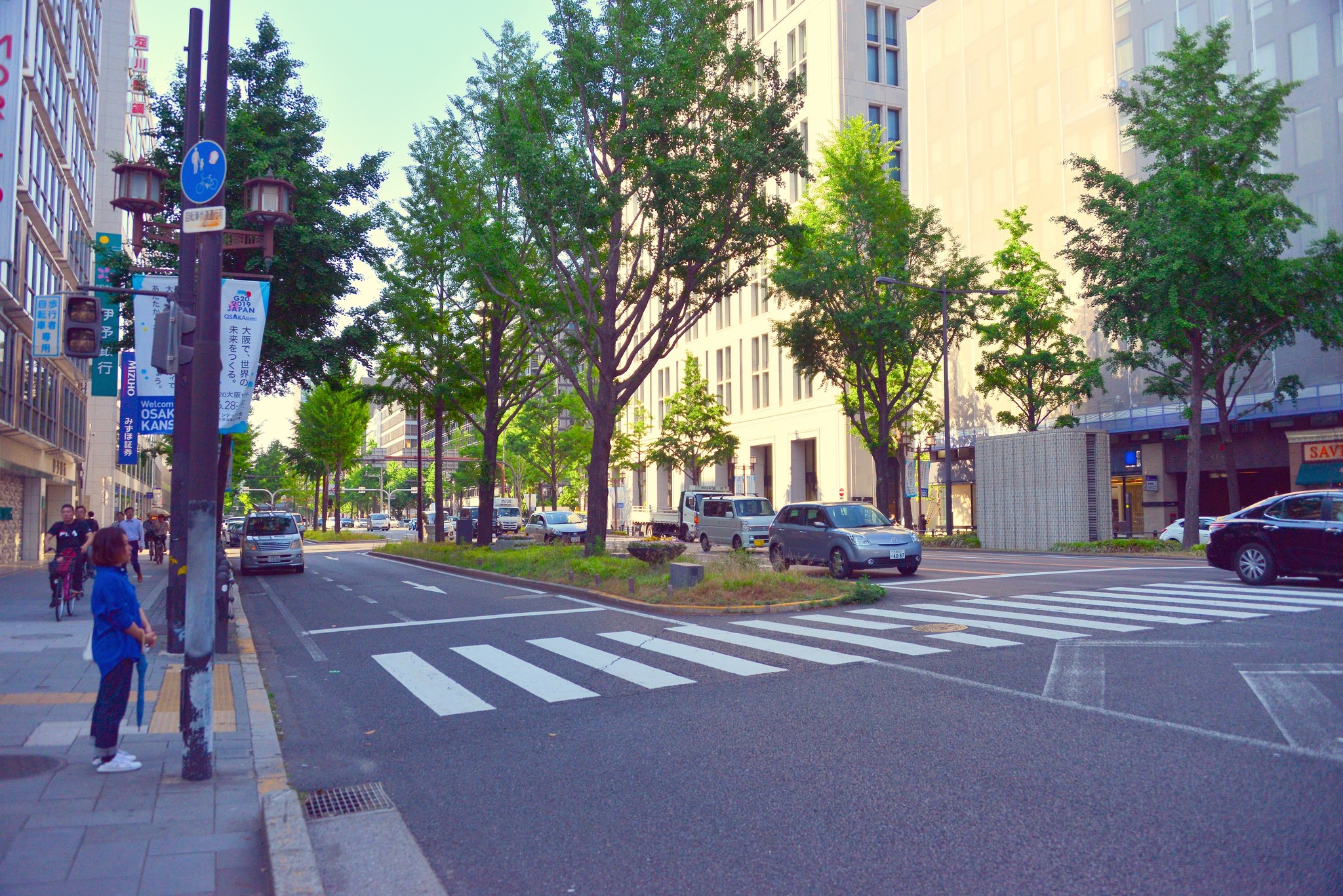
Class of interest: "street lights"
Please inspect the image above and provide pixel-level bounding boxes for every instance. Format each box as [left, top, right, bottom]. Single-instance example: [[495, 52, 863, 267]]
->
[[875, 270, 1009, 537], [730, 454, 757, 496], [112, 155, 297, 281], [607, 472, 625, 530], [900, 430, 936, 534]]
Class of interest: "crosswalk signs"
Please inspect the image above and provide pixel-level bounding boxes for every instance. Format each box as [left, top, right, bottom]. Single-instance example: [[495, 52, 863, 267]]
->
[[180, 140, 227, 205]]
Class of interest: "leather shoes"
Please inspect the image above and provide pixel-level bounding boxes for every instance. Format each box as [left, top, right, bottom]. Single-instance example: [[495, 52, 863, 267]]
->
[[137, 571, 142, 583]]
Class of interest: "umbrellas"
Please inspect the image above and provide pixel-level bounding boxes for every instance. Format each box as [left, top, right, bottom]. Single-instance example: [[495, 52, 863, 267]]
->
[[145, 508, 170, 518]]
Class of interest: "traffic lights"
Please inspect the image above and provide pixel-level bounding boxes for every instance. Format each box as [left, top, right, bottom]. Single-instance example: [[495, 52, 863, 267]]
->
[[246, 475, 256, 479], [405, 479, 415, 483], [64, 292, 104, 356], [151, 303, 197, 371]]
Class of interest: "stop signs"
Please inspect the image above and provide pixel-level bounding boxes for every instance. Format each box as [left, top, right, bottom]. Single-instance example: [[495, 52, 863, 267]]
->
[[839, 488, 845, 497], [333, 487, 335, 492]]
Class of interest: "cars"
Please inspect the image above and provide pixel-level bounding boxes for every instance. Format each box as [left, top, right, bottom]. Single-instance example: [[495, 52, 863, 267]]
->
[[448, 516, 459, 531], [524, 510, 587, 543], [313, 514, 417, 531], [1159, 516, 1218, 544], [1206, 489, 1343, 586]]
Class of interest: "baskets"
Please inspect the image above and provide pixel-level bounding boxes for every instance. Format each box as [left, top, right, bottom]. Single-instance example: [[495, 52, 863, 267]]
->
[[48, 556, 77, 576]]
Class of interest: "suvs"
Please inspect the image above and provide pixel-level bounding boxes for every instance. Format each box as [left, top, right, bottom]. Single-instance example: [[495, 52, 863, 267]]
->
[[460, 506, 503, 538], [769, 501, 922, 578]]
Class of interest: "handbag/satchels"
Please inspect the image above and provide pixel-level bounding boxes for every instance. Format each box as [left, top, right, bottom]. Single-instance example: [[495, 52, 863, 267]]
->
[[152, 534, 156, 541]]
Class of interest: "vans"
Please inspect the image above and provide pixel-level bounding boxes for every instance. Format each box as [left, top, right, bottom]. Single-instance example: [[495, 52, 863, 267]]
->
[[698, 493, 777, 552], [221, 511, 304, 573], [420, 511, 454, 540]]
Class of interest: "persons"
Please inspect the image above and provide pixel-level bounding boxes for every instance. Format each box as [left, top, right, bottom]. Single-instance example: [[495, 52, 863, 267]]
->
[[87, 526, 159, 772], [620, 516, 625, 531], [43, 501, 170, 608], [888, 513, 899, 526], [917, 514, 927, 534]]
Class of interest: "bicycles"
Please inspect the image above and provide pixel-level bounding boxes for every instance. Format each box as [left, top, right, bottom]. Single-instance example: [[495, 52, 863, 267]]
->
[[144, 534, 167, 565], [44, 547, 86, 622], [81, 557, 98, 582]]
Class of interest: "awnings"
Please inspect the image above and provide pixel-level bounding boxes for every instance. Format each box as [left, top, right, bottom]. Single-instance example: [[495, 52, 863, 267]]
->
[[1295, 462, 1343, 486]]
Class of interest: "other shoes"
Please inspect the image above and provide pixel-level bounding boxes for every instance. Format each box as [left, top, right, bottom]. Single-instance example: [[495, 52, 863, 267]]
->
[[164, 546, 167, 551], [145, 544, 149, 549], [153, 555, 158, 561]]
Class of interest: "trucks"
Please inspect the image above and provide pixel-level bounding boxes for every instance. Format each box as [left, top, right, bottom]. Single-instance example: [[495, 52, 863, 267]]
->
[[631, 486, 734, 542], [493, 497, 522, 534]]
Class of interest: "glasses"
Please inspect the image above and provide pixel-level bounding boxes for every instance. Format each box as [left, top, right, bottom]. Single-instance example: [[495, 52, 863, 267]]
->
[[158, 516, 164, 518], [76, 510, 85, 514]]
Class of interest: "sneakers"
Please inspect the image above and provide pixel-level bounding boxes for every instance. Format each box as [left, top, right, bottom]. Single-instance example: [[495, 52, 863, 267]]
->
[[49, 598, 57, 609], [95, 753, 143, 772], [92, 749, 137, 767], [69, 586, 84, 594], [86, 570, 97, 579]]
